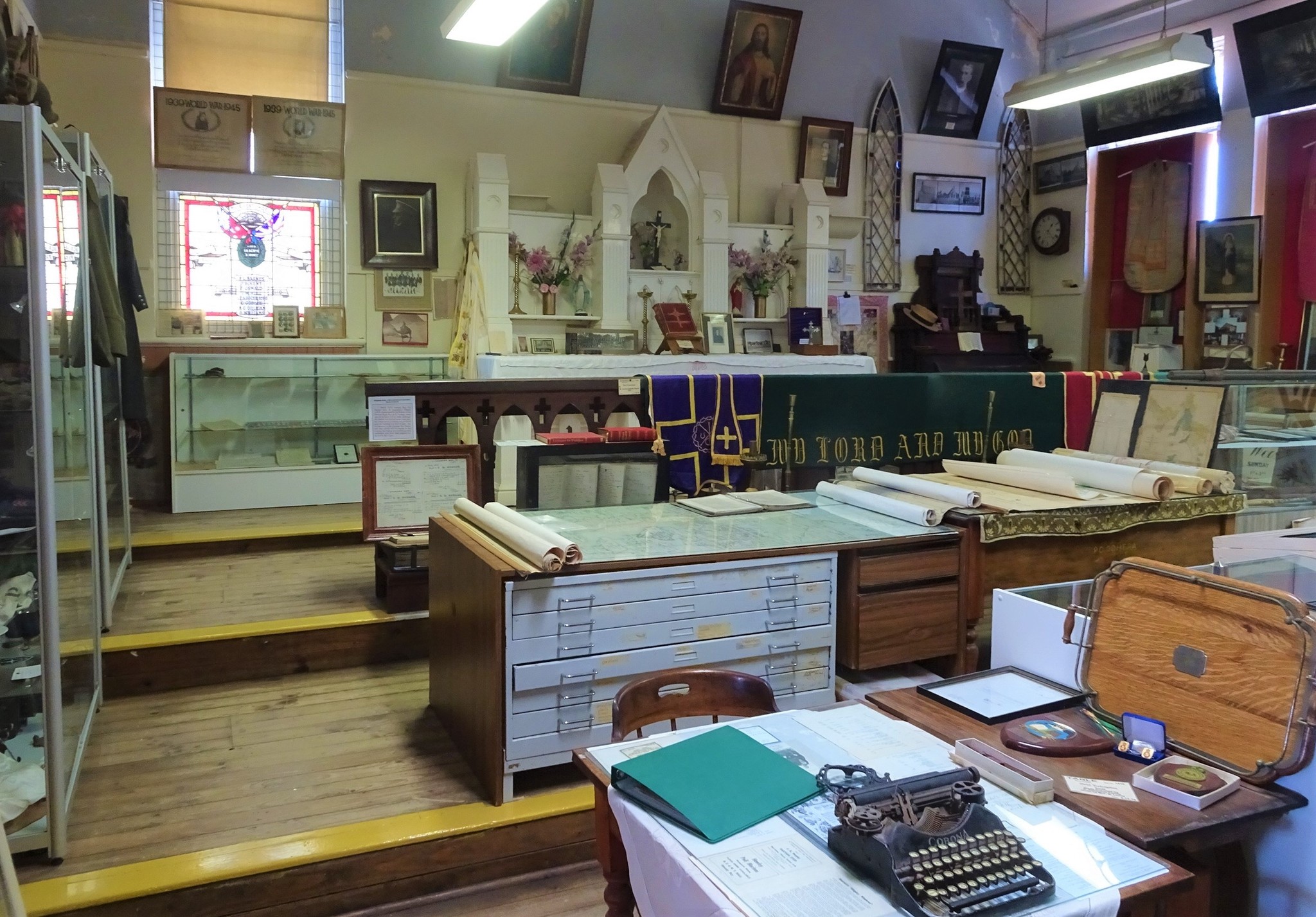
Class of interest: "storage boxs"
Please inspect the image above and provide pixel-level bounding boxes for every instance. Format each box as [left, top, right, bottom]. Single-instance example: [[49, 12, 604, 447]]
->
[[1113, 710, 1168, 766], [565, 673, 1312, 917], [948, 735, 1055, 806], [1130, 754, 1241, 812]]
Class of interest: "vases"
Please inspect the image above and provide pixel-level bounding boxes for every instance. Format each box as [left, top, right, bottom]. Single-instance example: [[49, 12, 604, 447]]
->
[[754, 296, 767, 318], [542, 290, 556, 315], [643, 253, 654, 269], [675, 264, 681, 270]]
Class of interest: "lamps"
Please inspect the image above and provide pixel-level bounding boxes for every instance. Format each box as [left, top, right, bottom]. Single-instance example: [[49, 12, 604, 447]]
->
[[438, 0, 551, 48], [1003, 0, 1214, 111]]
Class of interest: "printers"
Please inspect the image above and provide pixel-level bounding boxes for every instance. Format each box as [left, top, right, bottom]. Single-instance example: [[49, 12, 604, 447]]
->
[[816, 763, 1056, 917]]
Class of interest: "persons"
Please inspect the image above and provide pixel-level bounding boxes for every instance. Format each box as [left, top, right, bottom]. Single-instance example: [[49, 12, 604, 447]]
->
[[651, 224, 667, 248], [730, 277, 744, 314], [714, 329, 723, 343], [937, 62, 975, 115], [812, 141, 836, 179], [1217, 233, 1236, 285], [570, 273, 592, 312], [384, 199, 420, 252]]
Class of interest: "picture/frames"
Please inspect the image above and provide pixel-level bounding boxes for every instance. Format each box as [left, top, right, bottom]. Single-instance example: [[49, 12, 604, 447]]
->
[[710, 0, 1005, 216], [1032, 0, 1316, 195], [700, 314, 736, 354], [1195, 215, 1263, 305], [360, 178, 439, 312], [515, 334, 555, 354], [496, 0, 594, 96]]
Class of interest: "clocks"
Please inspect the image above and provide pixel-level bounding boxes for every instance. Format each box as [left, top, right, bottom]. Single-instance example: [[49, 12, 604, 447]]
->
[[1031, 206, 1072, 256]]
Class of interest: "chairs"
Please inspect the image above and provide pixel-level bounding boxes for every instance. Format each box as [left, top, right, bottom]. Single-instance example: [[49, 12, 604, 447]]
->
[[609, 664, 783, 745]]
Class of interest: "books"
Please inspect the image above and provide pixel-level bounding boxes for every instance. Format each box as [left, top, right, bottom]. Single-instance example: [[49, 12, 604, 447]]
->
[[651, 303, 704, 341], [670, 490, 818, 516], [597, 426, 657, 442], [536, 431, 605, 445]]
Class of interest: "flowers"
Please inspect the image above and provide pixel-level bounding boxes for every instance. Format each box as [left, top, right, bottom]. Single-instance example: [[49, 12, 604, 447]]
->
[[508, 210, 602, 294], [728, 229, 800, 299], [630, 221, 688, 265]]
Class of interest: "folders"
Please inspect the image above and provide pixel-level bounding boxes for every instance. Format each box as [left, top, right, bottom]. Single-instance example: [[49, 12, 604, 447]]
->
[[610, 725, 829, 844]]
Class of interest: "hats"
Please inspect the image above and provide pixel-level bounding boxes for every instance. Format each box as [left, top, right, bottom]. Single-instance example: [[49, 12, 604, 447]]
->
[[904, 304, 942, 332]]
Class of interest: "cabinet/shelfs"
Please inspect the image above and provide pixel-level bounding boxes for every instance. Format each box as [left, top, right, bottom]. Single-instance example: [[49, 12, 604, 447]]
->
[[0, 101, 133, 866], [430, 474, 969, 808], [166, 353, 450, 513]]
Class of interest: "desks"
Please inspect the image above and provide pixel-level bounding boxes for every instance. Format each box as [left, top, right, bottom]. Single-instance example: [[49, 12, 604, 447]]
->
[[948, 487, 1247, 671]]
